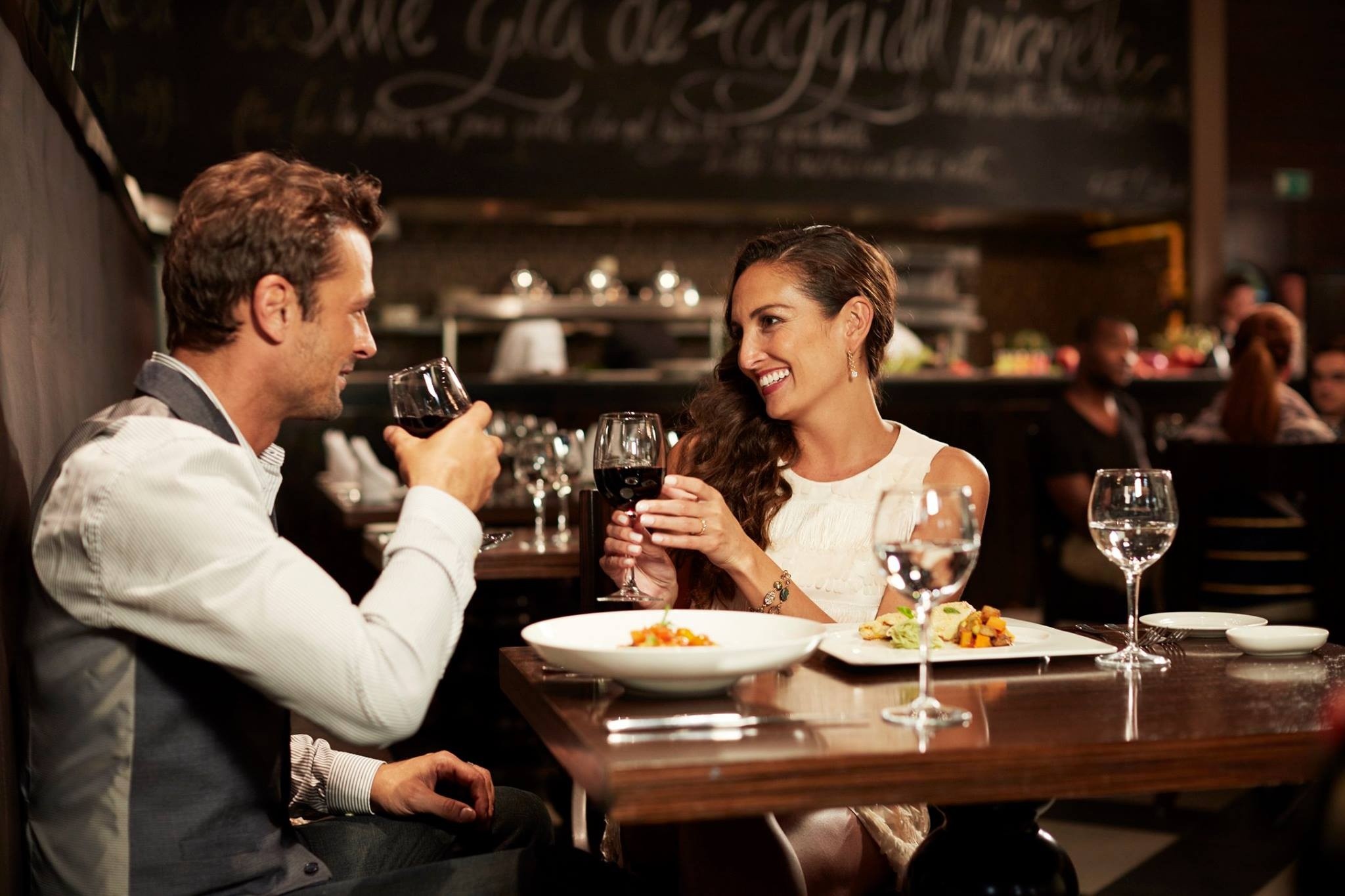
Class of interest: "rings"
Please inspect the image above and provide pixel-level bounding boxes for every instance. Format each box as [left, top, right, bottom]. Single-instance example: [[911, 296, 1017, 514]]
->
[[690, 519, 707, 537]]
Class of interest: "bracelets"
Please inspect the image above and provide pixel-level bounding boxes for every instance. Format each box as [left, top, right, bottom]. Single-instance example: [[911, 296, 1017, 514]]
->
[[748, 570, 791, 612], [768, 577, 792, 615]]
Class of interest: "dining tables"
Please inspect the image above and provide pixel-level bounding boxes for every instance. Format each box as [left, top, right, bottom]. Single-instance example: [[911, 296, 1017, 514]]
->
[[497, 624, 1345, 896], [361, 512, 590, 586], [311, 471, 410, 526]]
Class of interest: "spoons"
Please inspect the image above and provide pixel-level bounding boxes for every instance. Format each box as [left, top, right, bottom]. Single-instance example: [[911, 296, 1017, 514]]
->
[[541, 664, 568, 673]]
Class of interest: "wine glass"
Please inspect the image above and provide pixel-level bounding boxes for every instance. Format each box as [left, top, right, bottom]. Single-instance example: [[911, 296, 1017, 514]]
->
[[594, 410, 667, 601], [388, 356, 514, 556], [513, 435, 562, 551], [540, 431, 590, 552], [1088, 469, 1178, 669]]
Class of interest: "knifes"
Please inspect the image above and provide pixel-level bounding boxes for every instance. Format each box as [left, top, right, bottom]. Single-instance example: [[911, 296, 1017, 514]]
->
[[872, 481, 981, 726], [604, 710, 869, 733]]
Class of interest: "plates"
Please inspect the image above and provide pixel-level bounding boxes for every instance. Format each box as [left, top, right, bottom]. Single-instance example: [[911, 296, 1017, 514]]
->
[[1225, 625, 1331, 659], [1139, 612, 1269, 639], [519, 610, 827, 696], [818, 617, 1118, 667]]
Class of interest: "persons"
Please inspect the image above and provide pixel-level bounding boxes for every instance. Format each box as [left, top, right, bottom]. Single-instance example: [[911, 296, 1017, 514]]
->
[[1150, 260, 1331, 375], [1182, 303, 1342, 622], [598, 222, 991, 896], [11, 152, 615, 894], [1307, 343, 1345, 440], [1037, 312, 1162, 595]]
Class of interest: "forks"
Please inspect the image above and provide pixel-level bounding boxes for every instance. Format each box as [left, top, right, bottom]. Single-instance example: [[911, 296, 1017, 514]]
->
[[1074, 623, 1168, 646], [1104, 624, 1193, 645]]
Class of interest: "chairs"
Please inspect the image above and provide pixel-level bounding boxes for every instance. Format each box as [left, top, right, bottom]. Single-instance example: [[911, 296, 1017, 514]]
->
[[1156, 424, 1345, 635], [0, 413, 57, 896]]
